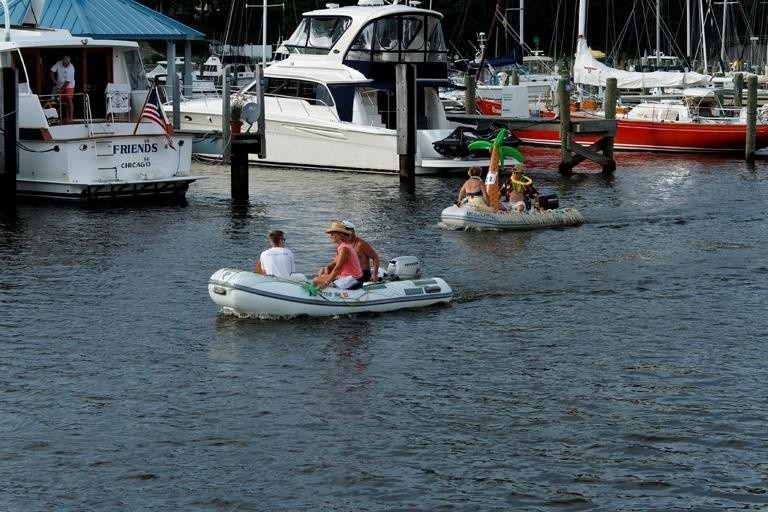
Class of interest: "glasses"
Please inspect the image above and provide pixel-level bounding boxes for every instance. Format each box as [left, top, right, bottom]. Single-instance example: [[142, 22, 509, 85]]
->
[[281, 238, 286, 241]]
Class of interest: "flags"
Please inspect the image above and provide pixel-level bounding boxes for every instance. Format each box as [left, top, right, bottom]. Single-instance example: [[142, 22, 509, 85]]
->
[[140, 86, 172, 135]]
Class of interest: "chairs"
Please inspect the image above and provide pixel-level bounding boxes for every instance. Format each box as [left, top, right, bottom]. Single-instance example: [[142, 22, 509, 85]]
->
[[18, 83, 60, 125], [104, 83, 132, 123]]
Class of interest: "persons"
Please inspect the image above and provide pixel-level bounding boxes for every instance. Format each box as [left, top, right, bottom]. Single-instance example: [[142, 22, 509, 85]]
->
[[729, 57, 739, 71], [458, 165, 489, 206], [317, 220, 380, 284], [48, 53, 76, 122], [311, 222, 364, 291], [256, 229, 308, 284], [497, 165, 539, 213]]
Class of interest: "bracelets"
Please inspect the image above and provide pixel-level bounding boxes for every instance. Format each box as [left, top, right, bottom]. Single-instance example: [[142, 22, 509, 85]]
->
[[372, 271, 378, 275], [325, 281, 328, 285]]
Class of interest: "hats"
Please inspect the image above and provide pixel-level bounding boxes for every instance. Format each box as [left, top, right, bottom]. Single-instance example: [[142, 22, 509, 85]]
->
[[514, 165, 524, 172], [325, 221, 356, 234]]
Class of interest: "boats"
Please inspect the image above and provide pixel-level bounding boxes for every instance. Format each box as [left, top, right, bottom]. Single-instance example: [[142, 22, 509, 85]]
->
[[207, 256, 454, 318], [440, 199, 584, 232]]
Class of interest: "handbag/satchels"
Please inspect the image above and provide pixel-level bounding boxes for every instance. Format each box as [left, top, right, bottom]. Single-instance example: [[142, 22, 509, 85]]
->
[[333, 275, 357, 290]]
